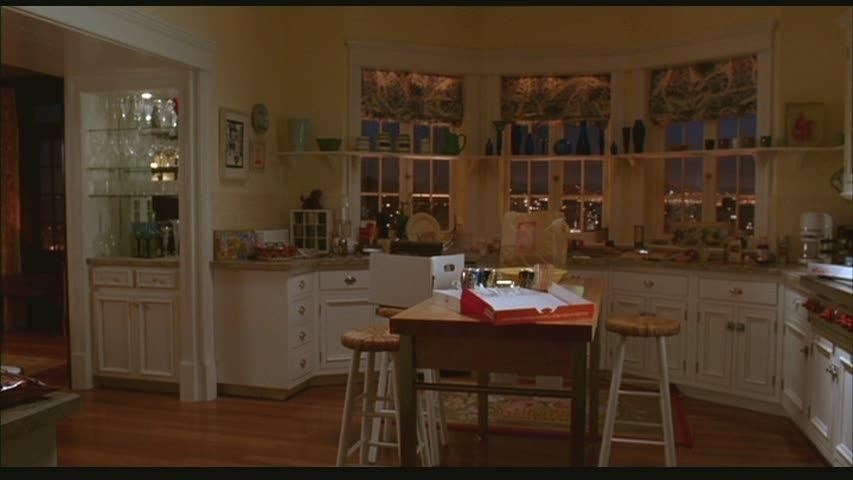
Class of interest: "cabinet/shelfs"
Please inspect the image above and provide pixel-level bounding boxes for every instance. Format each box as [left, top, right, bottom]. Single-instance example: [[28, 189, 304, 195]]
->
[[87, 121, 178, 197], [90, 258, 182, 391], [210, 257, 319, 403], [317, 263, 369, 383], [840, 7, 853, 201], [567, 270, 853, 467]]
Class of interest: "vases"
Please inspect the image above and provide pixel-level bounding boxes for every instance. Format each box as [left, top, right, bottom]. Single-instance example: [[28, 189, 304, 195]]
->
[[486, 120, 645, 155], [288, 118, 312, 152]]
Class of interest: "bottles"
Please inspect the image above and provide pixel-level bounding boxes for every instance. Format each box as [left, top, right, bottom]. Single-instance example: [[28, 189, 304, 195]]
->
[[755, 231, 792, 265], [355, 130, 468, 156], [482, 115, 648, 156]]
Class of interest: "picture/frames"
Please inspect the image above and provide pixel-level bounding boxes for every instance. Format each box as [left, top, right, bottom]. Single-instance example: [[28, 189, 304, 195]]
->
[[785, 102, 825, 146], [219, 107, 250, 181], [250, 143, 266, 169]]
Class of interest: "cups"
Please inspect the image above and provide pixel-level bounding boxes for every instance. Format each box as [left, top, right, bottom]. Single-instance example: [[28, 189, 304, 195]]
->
[[703, 135, 773, 150]]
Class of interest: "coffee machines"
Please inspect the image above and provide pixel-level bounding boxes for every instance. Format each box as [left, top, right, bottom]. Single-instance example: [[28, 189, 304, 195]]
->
[[798, 212, 834, 267]]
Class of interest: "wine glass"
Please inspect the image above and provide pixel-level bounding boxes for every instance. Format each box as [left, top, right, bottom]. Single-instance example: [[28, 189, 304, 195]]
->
[[86, 95, 178, 195]]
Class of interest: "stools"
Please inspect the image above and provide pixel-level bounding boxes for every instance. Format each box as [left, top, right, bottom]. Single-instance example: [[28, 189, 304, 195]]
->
[[336, 325, 428, 468], [369, 307, 449, 465]]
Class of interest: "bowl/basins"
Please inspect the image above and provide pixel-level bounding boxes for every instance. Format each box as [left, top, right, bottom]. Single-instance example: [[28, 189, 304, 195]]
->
[[317, 137, 343, 150]]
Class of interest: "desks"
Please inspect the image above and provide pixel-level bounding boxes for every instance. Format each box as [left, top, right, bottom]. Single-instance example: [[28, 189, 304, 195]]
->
[[0, 392, 81, 467], [390, 287, 603, 468]]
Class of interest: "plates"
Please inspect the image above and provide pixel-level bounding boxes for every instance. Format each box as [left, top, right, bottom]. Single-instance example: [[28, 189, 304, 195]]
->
[[405, 212, 441, 242]]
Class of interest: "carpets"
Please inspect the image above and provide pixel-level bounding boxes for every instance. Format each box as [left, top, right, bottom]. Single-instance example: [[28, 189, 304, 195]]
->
[[382, 377, 694, 449]]
